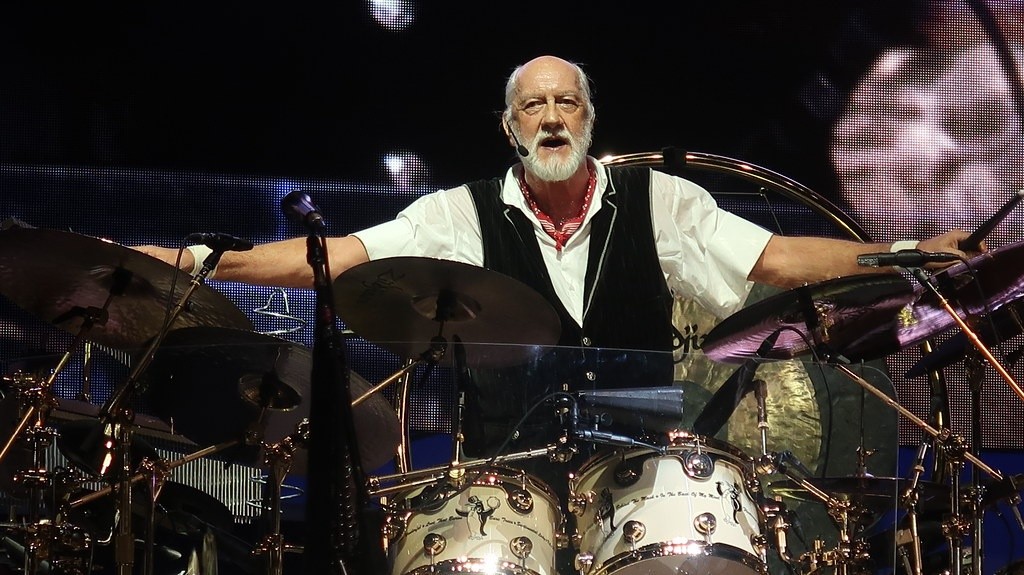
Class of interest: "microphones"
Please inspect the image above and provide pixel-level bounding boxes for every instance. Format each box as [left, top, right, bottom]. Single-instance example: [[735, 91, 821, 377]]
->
[[857, 248, 960, 267], [691, 331, 782, 440], [506, 122, 529, 157], [279, 190, 325, 231], [451, 335, 474, 389], [189, 233, 254, 252], [565, 401, 578, 452]]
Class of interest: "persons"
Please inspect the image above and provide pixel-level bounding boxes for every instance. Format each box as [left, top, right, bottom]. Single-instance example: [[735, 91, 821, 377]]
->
[[128, 56, 989, 480]]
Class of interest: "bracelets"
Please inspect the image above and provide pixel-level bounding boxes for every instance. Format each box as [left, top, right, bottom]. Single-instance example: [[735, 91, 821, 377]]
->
[[186, 245, 218, 282], [891, 240, 921, 267]]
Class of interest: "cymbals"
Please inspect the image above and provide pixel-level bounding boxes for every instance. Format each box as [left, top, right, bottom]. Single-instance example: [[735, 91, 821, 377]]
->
[[134, 326, 403, 476], [155, 478, 235, 538], [58, 426, 159, 482], [701, 272, 912, 365], [896, 239, 1024, 345], [903, 297, 1024, 380], [1, 227, 255, 352], [332, 256, 563, 370]]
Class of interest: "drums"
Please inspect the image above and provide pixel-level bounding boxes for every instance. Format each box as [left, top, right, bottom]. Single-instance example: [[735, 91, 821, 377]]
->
[[569, 440, 769, 575], [382, 461, 564, 575]]
